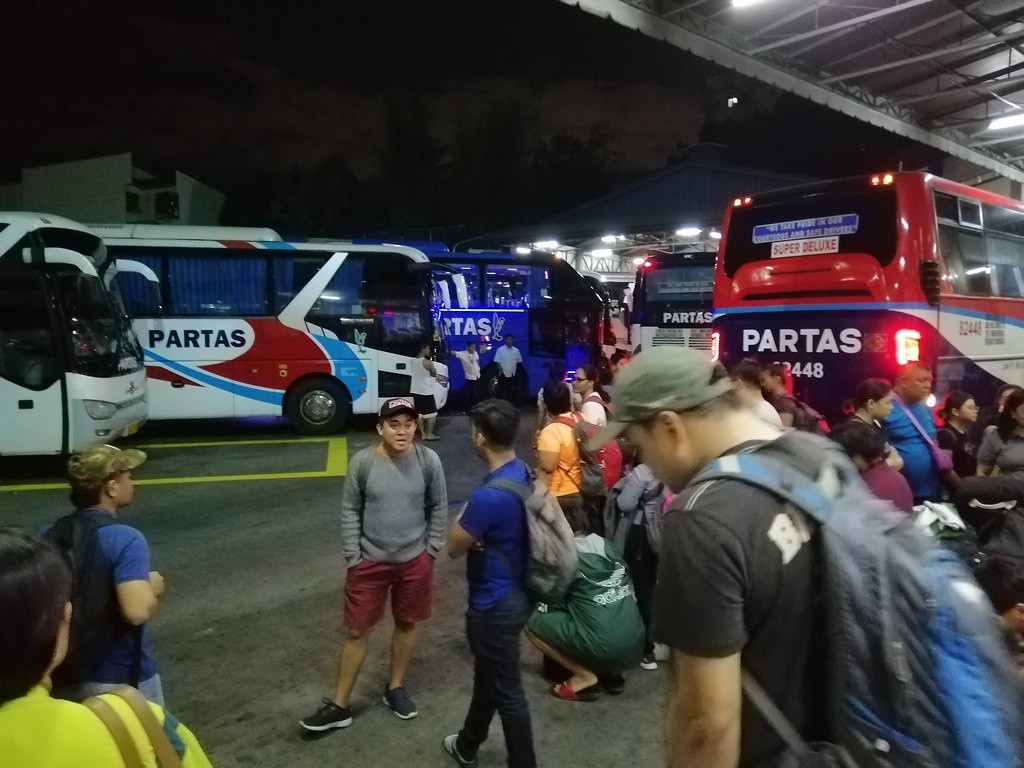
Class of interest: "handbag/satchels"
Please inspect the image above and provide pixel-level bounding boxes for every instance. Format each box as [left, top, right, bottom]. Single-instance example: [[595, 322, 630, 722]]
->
[[931, 442, 953, 471]]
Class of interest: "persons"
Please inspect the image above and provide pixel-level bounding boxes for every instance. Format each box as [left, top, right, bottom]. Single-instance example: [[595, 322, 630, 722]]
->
[[300, 398, 448, 731], [494, 335, 523, 399], [736, 352, 1024, 633], [443, 400, 538, 768], [535, 352, 638, 536], [0, 329, 51, 384], [0, 524, 213, 768], [583, 343, 824, 768], [41, 444, 165, 704], [452, 341, 481, 406], [525, 505, 645, 702], [617, 450, 658, 672], [410, 344, 440, 439]]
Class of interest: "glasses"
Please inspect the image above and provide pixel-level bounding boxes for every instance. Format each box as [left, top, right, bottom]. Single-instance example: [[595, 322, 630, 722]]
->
[[573, 374, 590, 382], [958, 406, 979, 410]]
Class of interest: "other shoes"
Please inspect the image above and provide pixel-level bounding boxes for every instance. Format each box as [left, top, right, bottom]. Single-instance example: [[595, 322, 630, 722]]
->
[[640, 651, 657, 670], [654, 639, 670, 661]]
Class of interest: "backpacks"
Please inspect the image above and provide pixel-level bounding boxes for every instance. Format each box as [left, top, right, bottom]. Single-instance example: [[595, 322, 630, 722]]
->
[[549, 410, 623, 496], [782, 396, 831, 438], [693, 431, 1023, 768], [486, 458, 579, 597], [580, 397, 634, 466], [40, 516, 126, 691]]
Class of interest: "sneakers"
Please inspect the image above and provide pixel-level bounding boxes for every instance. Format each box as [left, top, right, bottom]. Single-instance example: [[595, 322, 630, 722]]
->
[[298, 697, 352, 730], [443, 734, 479, 768], [383, 682, 418, 720]]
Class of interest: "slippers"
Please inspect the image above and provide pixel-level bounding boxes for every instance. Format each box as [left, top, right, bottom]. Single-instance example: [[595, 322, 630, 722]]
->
[[602, 674, 624, 693], [551, 680, 599, 701]]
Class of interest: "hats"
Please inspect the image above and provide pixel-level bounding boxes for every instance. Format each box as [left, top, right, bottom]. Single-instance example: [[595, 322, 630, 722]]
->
[[378, 398, 419, 422], [68, 444, 147, 492], [588, 346, 732, 451]]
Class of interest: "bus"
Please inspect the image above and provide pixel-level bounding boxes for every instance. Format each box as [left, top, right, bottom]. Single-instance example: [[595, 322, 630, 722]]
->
[[712, 170, 1024, 427], [295, 238, 612, 409], [0, 212, 160, 458], [82, 222, 469, 433], [630, 251, 718, 361], [579, 269, 636, 365]]
